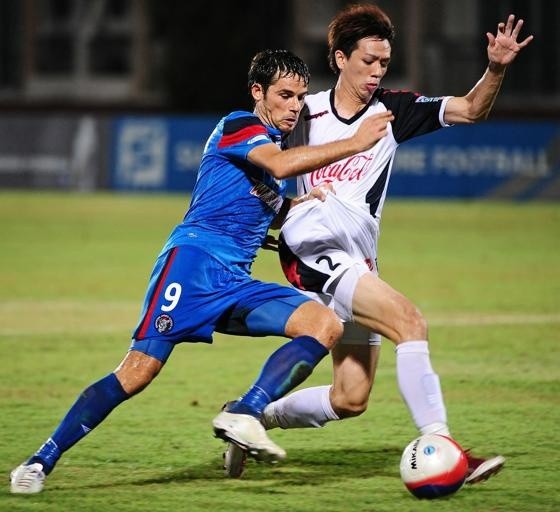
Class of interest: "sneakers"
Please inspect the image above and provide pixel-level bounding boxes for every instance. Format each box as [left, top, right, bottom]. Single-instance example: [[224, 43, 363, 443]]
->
[[10, 460, 46, 494], [222, 401, 247, 478], [213, 412, 286, 464], [464, 448, 505, 484]]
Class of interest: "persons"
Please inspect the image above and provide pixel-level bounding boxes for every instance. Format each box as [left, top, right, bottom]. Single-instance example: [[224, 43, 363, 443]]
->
[[221, 2, 534, 485], [8, 48, 395, 496]]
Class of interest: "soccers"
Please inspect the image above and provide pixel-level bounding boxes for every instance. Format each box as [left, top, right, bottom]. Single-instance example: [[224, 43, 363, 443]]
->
[[399, 435, 468, 499]]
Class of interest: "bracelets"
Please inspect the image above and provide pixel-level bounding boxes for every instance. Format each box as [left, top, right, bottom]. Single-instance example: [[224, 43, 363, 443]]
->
[[487, 60, 507, 75]]
